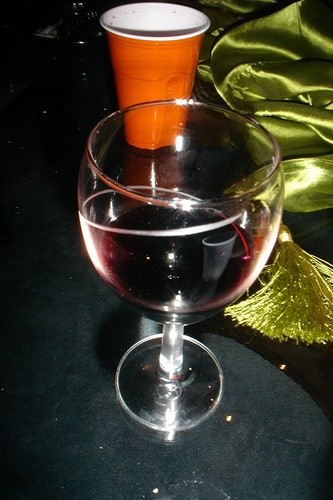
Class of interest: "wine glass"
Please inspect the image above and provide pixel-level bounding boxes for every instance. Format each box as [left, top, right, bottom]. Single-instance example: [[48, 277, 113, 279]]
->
[[77, 99, 285, 432]]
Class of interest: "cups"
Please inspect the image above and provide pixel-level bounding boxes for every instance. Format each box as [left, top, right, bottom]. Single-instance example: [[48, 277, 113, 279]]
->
[[100, 3, 211, 151]]
